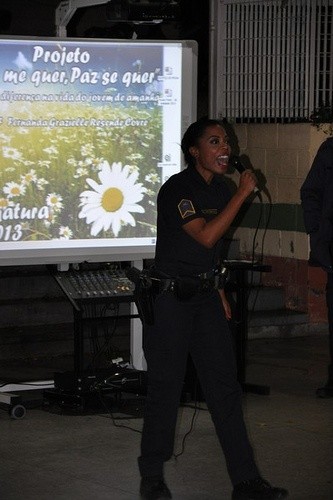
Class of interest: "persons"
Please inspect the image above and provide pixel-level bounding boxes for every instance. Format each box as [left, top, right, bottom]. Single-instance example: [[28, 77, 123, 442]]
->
[[300, 136, 333, 398], [138, 117, 290, 500]]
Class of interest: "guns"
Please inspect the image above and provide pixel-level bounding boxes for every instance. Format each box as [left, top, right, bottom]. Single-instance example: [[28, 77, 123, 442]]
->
[[125, 268, 151, 292]]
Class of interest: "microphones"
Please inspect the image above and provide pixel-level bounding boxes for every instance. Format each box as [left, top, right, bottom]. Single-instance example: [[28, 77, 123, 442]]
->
[[231, 153, 262, 202]]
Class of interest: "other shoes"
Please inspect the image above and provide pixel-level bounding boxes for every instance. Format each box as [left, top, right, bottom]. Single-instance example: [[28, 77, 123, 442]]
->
[[139, 473, 171, 500], [231, 476, 288, 500], [315, 388, 333, 398]]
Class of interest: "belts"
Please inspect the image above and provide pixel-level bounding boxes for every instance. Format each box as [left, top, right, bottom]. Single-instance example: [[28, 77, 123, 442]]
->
[[156, 279, 174, 291]]
